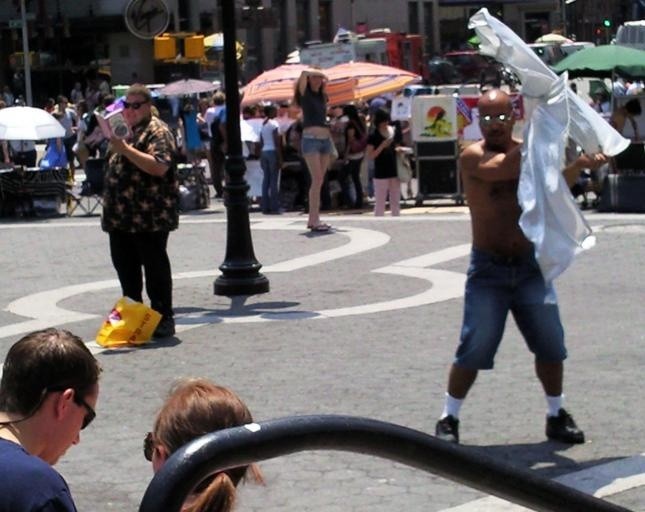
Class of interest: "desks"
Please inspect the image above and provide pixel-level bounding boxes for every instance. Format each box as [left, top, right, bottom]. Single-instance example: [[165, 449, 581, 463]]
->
[[0, 168, 68, 216]]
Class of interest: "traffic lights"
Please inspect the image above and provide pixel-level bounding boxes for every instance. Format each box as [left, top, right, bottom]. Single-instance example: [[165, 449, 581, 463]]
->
[[9, 20, 22, 28]]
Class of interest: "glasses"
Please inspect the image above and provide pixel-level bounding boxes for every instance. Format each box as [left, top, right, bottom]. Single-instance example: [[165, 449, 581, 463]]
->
[[144, 432, 170, 461], [476, 110, 517, 125], [122, 100, 147, 109], [73, 391, 95, 429]]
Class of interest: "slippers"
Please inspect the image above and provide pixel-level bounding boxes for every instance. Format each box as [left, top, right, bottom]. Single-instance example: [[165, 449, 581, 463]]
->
[[308, 221, 332, 233]]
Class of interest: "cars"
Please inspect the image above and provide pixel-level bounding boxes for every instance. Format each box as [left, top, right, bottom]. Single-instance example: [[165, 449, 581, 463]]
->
[[441, 51, 501, 91], [523, 42, 564, 68]]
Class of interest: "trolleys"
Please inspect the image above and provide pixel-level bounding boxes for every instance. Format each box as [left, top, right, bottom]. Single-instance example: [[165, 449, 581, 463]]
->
[[415, 135, 466, 210]]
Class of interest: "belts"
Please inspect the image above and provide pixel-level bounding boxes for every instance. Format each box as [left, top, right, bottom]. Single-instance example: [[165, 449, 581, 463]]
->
[[302, 134, 330, 141]]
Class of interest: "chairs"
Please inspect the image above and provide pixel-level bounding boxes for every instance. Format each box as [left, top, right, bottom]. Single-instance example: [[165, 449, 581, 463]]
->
[[64, 157, 106, 217]]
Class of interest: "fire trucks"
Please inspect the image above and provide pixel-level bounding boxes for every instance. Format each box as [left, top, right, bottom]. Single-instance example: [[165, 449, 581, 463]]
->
[[298, 23, 431, 88]]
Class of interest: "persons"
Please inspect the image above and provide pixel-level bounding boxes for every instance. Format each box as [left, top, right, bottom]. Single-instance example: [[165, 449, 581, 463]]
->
[[157, 94, 304, 214], [143, 374, 268, 511], [330, 95, 406, 218], [436, 87, 615, 448], [0, 80, 116, 183], [98, 85, 184, 343], [534, 19, 574, 47], [1, 327, 107, 511], [602, 98, 640, 170], [290, 68, 336, 231]]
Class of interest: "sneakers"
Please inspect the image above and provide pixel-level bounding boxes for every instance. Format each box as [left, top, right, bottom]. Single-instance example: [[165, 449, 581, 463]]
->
[[435, 415, 462, 444], [543, 408, 586, 445], [152, 316, 176, 337]]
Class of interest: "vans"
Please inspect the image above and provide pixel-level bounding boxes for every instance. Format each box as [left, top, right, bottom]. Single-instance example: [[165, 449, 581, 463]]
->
[[611, 20, 645, 53]]
